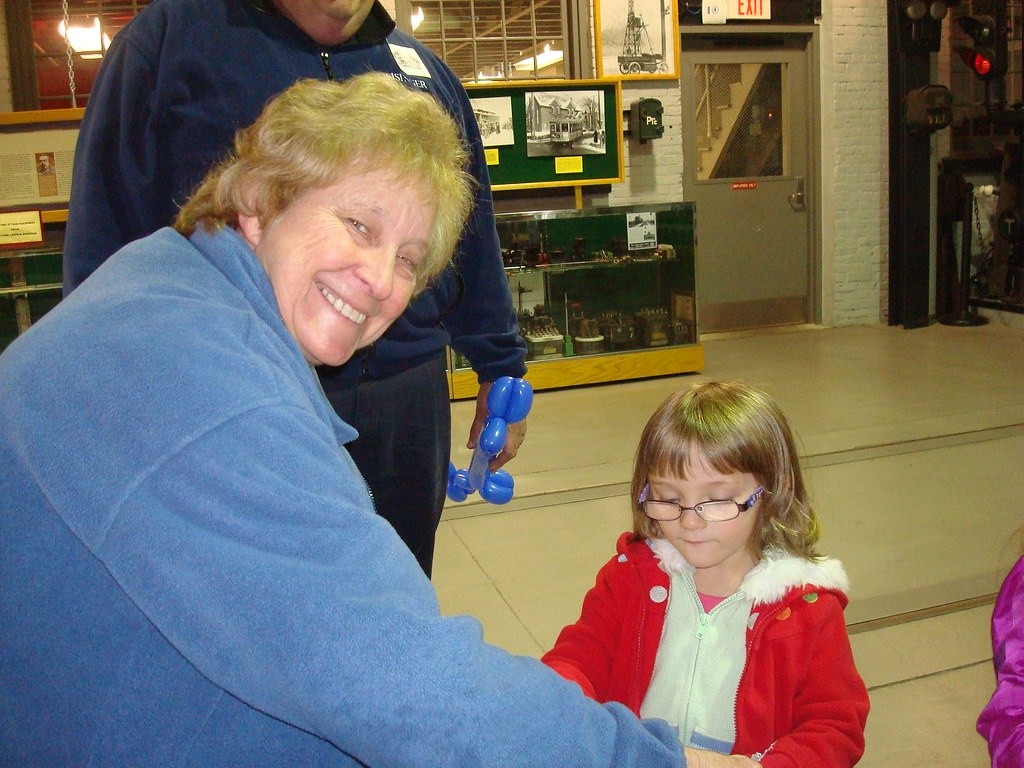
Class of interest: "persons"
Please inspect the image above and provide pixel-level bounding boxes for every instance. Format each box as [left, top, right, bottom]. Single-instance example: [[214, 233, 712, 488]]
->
[[0, 72, 760, 768], [540, 382, 870, 768], [64, 0, 526, 582]]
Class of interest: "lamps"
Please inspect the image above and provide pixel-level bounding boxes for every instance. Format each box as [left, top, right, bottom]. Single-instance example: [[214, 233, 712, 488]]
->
[[57, 15, 112, 59], [513, 39, 564, 71]]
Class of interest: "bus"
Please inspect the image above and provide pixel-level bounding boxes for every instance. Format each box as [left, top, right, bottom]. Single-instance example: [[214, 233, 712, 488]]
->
[[550, 118, 584, 145], [472, 108, 500, 134]]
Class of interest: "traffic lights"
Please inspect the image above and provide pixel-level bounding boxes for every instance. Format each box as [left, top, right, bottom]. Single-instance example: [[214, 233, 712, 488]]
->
[[958, 0, 1008, 83]]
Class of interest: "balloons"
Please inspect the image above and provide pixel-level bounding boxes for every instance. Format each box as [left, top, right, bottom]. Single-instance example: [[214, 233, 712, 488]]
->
[[447, 377, 533, 505]]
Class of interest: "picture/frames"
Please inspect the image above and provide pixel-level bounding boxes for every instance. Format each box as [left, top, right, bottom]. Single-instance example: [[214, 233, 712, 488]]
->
[[593, 0, 680, 83]]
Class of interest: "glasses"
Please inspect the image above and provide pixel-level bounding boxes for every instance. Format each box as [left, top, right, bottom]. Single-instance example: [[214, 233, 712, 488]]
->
[[638, 484, 766, 523]]
